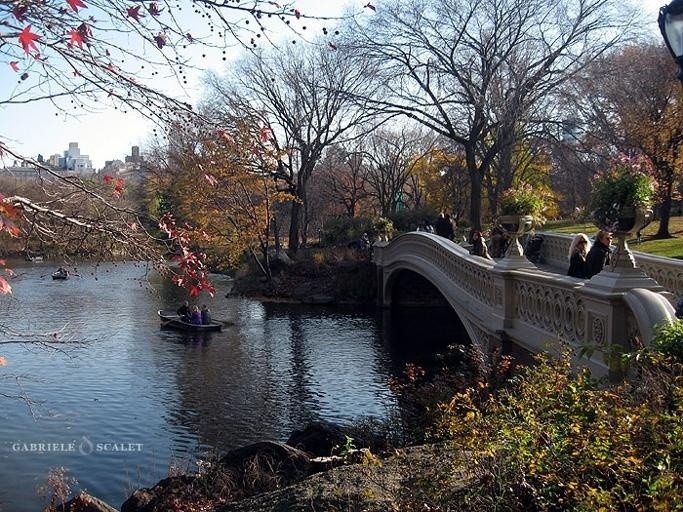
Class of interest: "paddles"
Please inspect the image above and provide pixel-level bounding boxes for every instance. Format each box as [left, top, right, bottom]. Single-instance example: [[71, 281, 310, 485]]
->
[[212, 319, 234, 325], [162, 315, 186, 327]]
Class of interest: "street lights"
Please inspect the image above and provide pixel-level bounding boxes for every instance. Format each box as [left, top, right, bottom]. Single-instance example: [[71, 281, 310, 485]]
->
[[653, 0, 683, 86], [318, 227, 320, 244]]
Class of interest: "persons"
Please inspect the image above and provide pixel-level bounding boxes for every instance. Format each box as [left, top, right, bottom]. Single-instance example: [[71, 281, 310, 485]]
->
[[176, 300, 190, 321], [191, 333, 200, 353], [188, 305, 201, 324], [584, 229, 613, 279], [449, 212, 457, 242], [566, 232, 592, 279], [468, 230, 493, 261], [439, 214, 453, 240], [201, 332, 212, 353], [200, 303, 212, 325], [435, 212, 444, 234]]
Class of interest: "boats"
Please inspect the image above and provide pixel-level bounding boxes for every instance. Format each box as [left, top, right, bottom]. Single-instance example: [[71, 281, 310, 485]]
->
[[50, 270, 69, 280], [154, 307, 225, 334]]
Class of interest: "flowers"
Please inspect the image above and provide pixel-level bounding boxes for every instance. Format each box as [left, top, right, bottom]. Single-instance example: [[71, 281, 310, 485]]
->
[[588, 149, 660, 232], [497, 183, 544, 215]]
